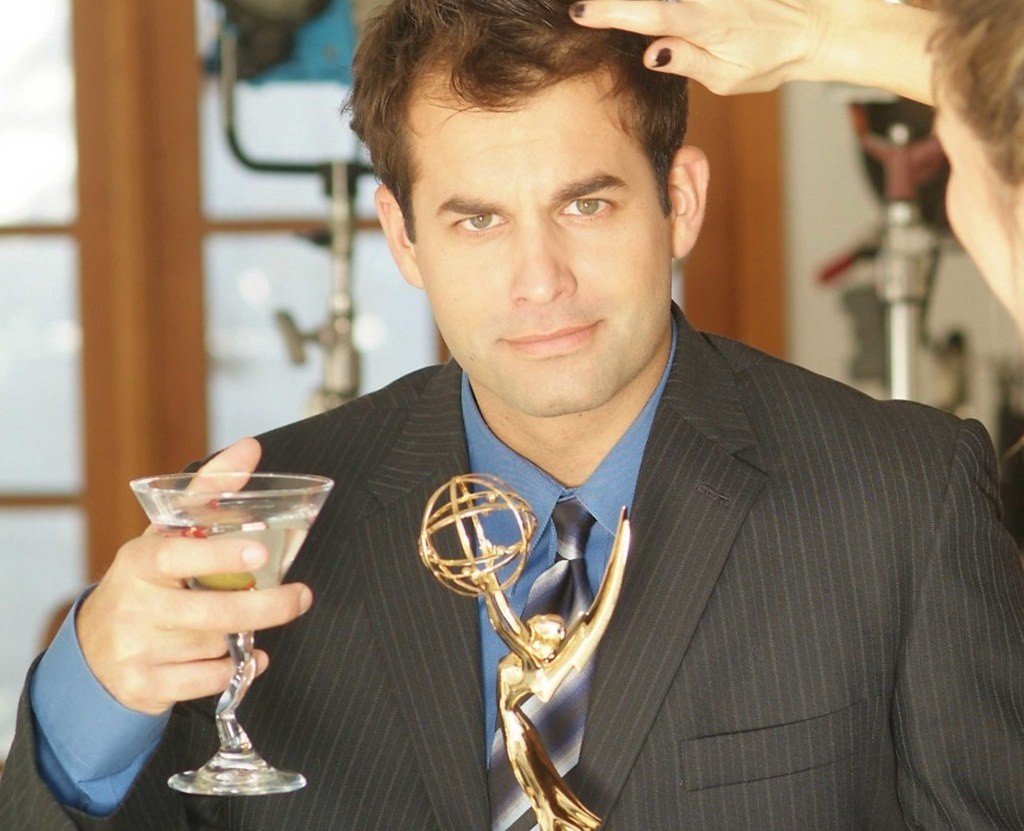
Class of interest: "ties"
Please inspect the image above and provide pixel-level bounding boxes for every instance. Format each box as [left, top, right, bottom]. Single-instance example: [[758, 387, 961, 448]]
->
[[486, 496, 597, 831]]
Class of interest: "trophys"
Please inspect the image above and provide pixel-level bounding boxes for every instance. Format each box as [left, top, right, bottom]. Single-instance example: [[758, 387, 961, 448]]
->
[[419, 471, 633, 830]]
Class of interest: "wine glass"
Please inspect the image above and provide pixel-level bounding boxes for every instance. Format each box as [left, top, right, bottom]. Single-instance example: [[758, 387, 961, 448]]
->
[[128, 473, 335, 796]]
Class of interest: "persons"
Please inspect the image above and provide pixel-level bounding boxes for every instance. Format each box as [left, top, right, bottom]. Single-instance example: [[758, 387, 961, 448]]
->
[[565, 0, 1024, 552], [0, 0, 1024, 831]]
